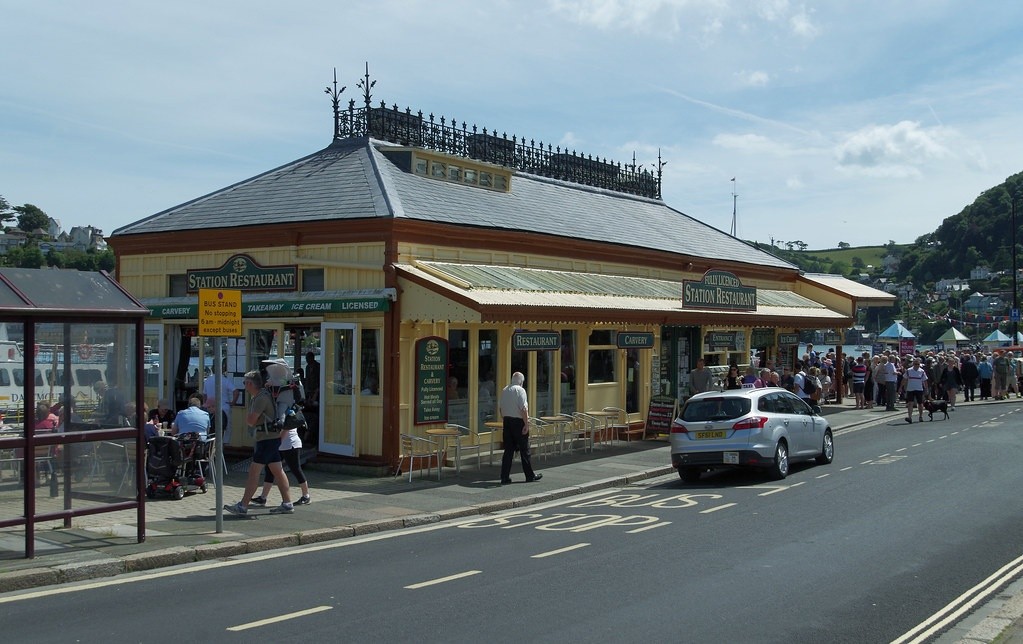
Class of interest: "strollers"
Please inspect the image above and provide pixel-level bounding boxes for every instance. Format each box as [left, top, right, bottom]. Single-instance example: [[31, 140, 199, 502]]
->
[[146, 432, 208, 499]]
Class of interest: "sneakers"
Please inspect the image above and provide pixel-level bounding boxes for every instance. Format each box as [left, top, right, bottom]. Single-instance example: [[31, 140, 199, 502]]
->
[[224, 502, 247, 515], [293, 496, 311, 505], [270, 503, 295, 513], [250, 496, 266, 505]]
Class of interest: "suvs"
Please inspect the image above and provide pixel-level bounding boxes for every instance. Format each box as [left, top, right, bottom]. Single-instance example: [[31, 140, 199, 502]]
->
[[669, 388, 835, 479]]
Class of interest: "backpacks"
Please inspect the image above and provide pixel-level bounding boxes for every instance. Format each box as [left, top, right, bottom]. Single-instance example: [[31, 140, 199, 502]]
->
[[798, 373, 817, 395]]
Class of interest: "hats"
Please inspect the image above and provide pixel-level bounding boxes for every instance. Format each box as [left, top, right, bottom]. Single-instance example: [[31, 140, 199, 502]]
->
[[93, 381, 104, 394], [949, 349, 954, 353], [824, 358, 833, 364], [906, 354, 914, 361], [158, 398, 170, 410], [828, 352, 836, 355]]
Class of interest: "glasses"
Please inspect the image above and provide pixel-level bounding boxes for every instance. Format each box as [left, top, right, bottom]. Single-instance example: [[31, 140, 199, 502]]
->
[[243, 380, 252, 385], [731, 368, 738, 371]]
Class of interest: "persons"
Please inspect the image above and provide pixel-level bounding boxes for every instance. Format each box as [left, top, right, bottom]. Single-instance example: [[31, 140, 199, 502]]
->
[[449, 345, 604, 401], [17, 352, 379, 517], [688, 342, 1023, 424], [500, 372, 543, 484]]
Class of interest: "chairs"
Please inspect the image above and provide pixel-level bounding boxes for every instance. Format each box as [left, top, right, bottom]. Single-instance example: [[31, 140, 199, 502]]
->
[[394, 432, 441, 484], [820, 382, 832, 405], [12, 416, 228, 498], [519, 406, 631, 464], [441, 424, 481, 474]]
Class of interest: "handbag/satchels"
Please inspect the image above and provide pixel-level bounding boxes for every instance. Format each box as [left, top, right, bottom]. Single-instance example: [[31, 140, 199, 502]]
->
[[283, 403, 306, 430]]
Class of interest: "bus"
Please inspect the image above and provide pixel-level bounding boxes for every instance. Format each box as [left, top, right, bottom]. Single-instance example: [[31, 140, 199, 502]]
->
[[0, 340, 226, 421]]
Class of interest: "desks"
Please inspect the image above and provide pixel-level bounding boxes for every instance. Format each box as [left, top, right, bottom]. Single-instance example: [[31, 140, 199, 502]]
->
[[423, 428, 461, 478], [539, 416, 571, 457], [585, 411, 619, 451], [484, 422, 503, 467]]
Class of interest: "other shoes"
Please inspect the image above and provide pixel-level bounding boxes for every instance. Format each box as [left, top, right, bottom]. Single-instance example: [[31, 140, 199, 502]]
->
[[501, 478, 511, 485], [830, 390, 1022, 423], [532, 473, 543, 480]]
